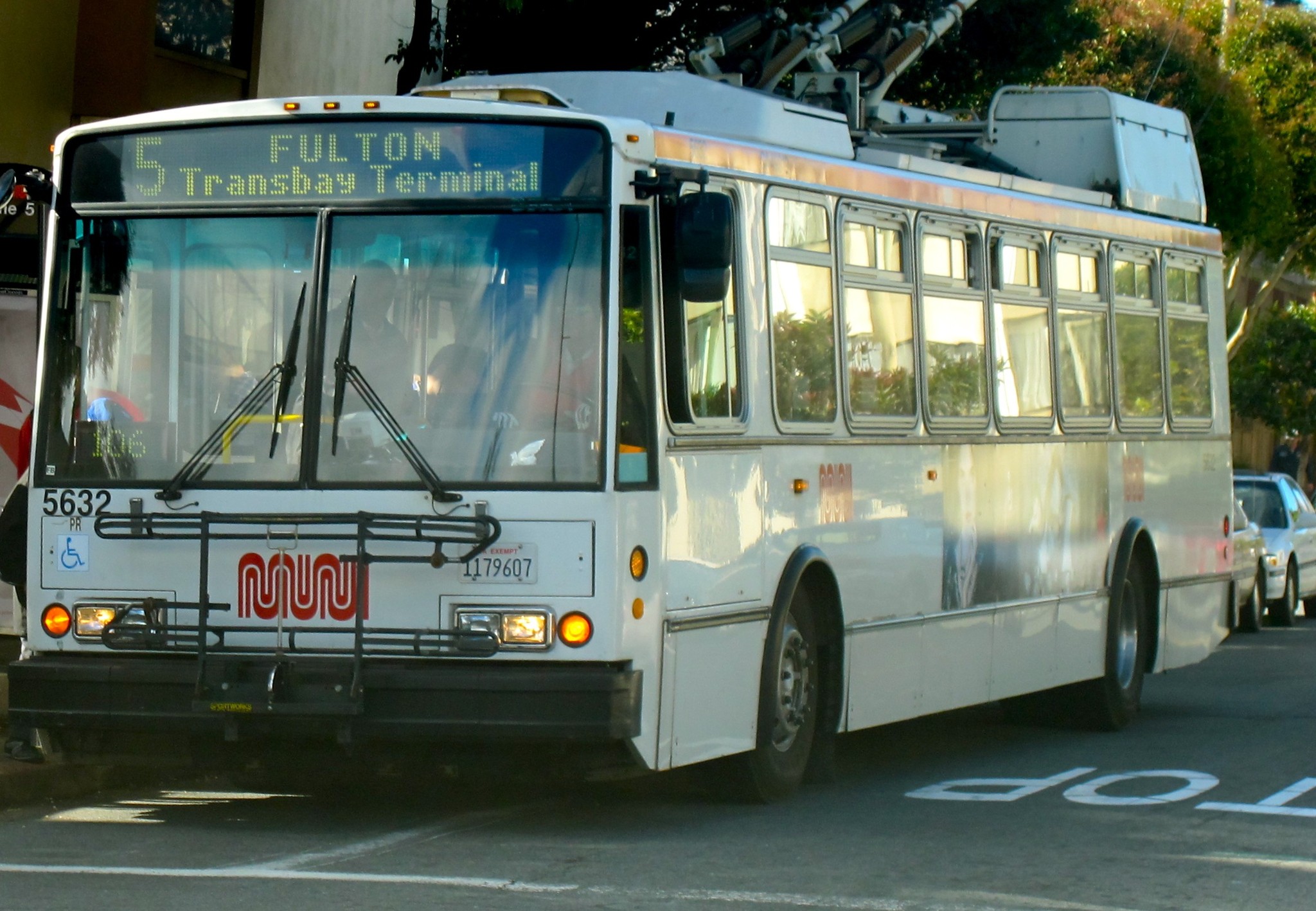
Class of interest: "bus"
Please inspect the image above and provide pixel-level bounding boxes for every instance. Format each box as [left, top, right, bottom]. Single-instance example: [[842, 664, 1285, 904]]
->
[[0, 0, 1238, 809]]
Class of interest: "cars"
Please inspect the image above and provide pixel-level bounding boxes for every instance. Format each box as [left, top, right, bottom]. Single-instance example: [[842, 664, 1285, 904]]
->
[[1231, 468, 1316, 627], [1230, 495, 1268, 632]]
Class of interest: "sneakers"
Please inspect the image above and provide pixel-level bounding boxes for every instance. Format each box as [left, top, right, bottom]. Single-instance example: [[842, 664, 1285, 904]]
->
[[2, 737, 41, 760]]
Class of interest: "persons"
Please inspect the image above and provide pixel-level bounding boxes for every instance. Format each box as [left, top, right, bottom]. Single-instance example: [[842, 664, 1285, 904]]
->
[[215, 258, 644, 475]]
